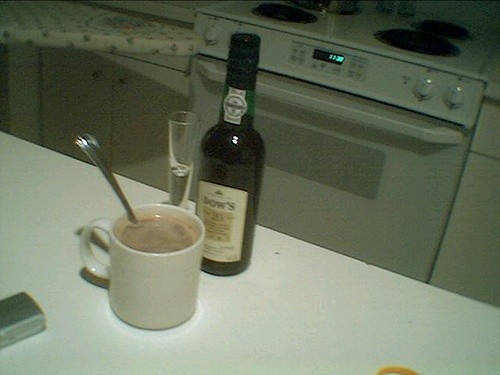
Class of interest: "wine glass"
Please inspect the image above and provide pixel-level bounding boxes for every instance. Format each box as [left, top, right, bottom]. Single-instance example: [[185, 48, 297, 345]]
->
[[168, 110, 200, 206]]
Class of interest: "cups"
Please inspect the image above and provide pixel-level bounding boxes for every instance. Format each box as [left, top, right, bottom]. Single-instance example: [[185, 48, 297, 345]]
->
[[78, 203, 205, 331]]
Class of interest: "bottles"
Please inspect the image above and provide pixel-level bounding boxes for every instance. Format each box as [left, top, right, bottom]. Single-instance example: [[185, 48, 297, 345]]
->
[[196, 32, 267, 276]]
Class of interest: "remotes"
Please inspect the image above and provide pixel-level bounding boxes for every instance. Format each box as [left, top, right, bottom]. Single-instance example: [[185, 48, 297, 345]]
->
[[1, 291, 46, 349]]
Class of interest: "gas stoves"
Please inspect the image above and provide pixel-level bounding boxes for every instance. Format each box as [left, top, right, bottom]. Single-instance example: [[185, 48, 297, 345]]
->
[[194, 0, 500, 131]]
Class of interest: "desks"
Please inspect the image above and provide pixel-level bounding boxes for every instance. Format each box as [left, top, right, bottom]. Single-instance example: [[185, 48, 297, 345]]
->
[[0, 132, 500, 371]]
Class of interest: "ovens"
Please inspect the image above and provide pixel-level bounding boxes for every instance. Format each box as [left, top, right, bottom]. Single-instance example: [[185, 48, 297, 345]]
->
[[188, 55, 469, 285]]
